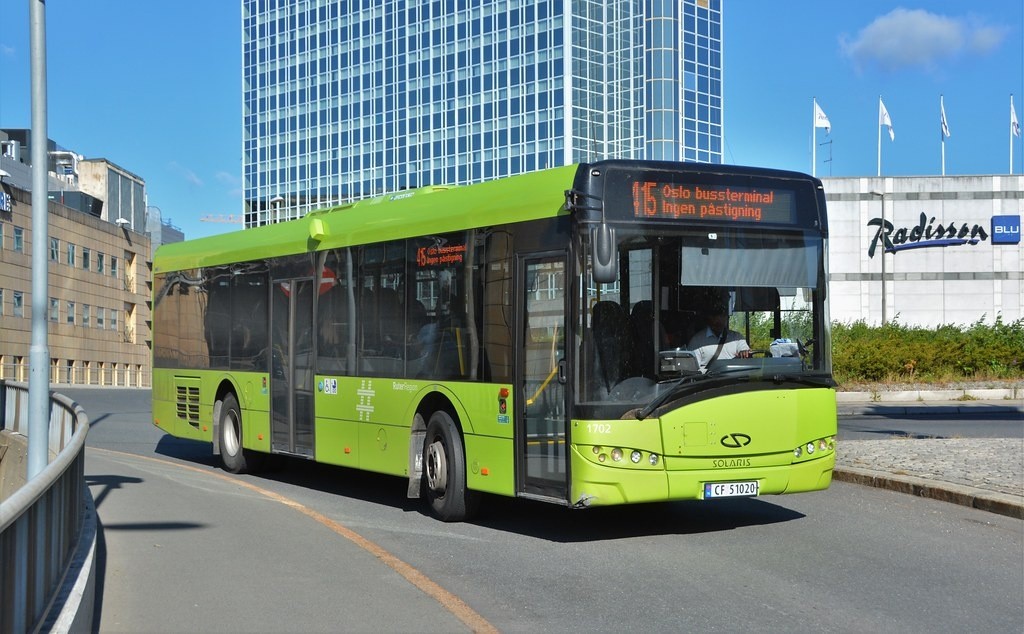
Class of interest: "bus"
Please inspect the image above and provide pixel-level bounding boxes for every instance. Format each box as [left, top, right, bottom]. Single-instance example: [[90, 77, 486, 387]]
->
[[150, 159, 839, 523]]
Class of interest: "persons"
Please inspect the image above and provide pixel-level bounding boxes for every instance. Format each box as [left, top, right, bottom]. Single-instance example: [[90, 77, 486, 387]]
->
[[686, 306, 752, 369]]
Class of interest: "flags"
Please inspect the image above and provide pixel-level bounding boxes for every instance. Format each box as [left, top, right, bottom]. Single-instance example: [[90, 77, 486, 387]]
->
[[1012, 103, 1021, 138], [815, 102, 831, 135], [940, 101, 950, 138], [880, 100, 894, 142]]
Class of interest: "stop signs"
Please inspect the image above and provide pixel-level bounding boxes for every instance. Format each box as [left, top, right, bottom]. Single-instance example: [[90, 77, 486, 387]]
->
[[318, 269, 335, 295]]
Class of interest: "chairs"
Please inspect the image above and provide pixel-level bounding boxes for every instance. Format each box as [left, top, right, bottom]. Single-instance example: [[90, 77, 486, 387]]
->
[[592, 301, 645, 396]]
